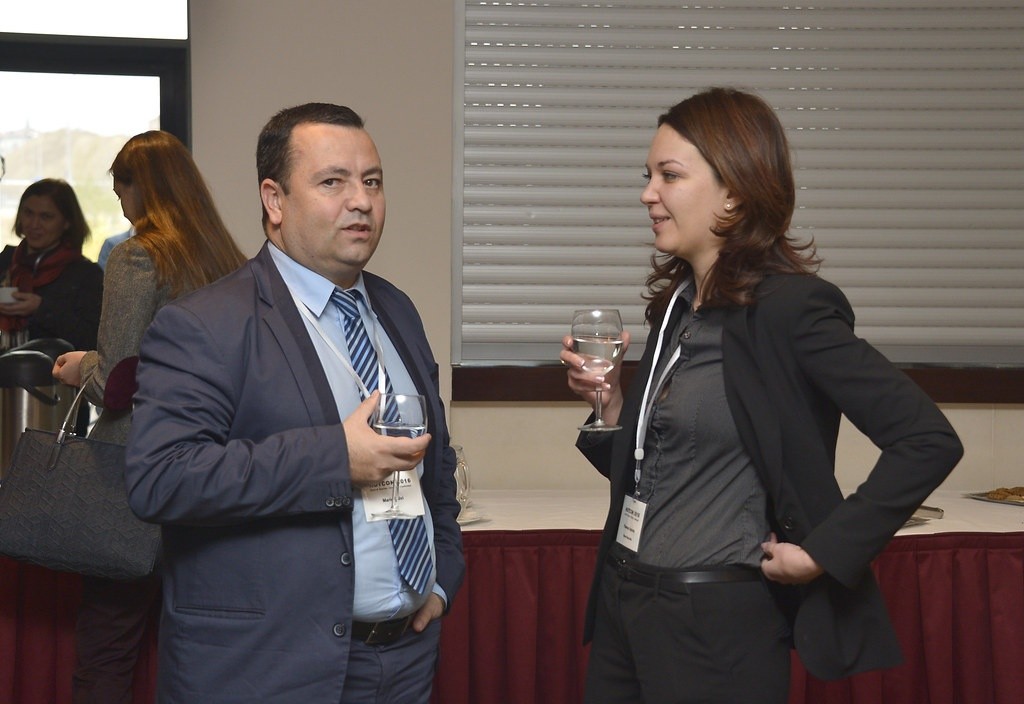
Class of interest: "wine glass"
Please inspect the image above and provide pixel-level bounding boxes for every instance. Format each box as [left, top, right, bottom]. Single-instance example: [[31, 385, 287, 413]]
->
[[570, 307, 625, 434], [370, 393, 428, 520]]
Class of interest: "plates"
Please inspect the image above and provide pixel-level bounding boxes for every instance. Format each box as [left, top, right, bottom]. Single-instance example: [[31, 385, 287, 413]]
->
[[457, 516, 481, 526]]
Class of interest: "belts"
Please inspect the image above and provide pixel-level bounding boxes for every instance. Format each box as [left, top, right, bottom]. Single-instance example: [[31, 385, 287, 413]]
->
[[607, 553, 768, 595], [349, 608, 422, 645]]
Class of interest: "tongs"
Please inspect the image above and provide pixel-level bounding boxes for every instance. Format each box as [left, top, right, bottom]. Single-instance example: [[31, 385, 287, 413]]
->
[[914, 504, 944, 519]]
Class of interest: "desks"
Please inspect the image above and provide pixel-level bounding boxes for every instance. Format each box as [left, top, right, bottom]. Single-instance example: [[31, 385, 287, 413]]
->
[[1, 482, 1024, 704]]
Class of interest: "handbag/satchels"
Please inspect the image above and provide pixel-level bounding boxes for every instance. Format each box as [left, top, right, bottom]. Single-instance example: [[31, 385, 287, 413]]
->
[[0, 374, 163, 579]]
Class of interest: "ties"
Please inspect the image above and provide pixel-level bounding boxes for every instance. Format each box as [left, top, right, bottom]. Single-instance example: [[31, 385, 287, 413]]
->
[[330, 288, 434, 595]]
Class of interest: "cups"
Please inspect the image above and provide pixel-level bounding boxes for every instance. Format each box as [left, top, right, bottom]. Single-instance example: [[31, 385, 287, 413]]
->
[[0, 287, 17, 303], [451, 444, 472, 519]]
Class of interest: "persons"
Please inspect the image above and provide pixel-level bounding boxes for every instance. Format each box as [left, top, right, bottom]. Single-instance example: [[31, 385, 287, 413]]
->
[[559, 86, 964, 704], [1, 180, 137, 437], [52, 130, 249, 704], [125, 101, 465, 704]]
